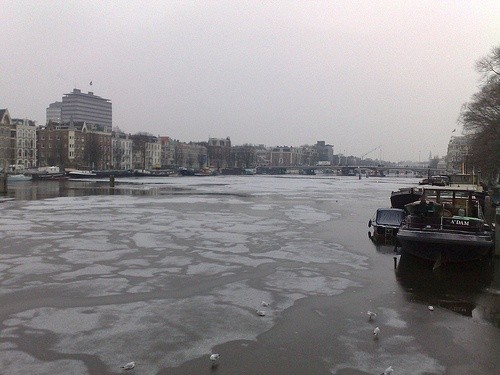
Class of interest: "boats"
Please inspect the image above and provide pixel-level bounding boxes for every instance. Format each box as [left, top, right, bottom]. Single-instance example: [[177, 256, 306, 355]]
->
[[32, 172, 67, 179], [397, 183, 495, 264], [69, 172, 96, 178], [193, 168, 213, 176], [367, 206, 402, 240], [429, 175, 448, 186], [391, 186, 423, 208], [134, 169, 169, 177], [242, 169, 257, 175]]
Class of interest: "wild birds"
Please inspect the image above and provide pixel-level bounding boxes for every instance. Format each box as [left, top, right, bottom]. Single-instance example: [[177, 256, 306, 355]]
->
[[367, 311, 378, 320], [256, 309, 267, 316], [373, 326, 380, 336], [428, 306, 434, 311], [380, 365, 395, 375], [209, 354, 223, 364], [120, 360, 136, 370], [261, 301, 270, 307]]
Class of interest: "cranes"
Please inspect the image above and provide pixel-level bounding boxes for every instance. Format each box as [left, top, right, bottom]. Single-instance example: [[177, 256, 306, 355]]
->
[[359, 145, 381, 160]]
